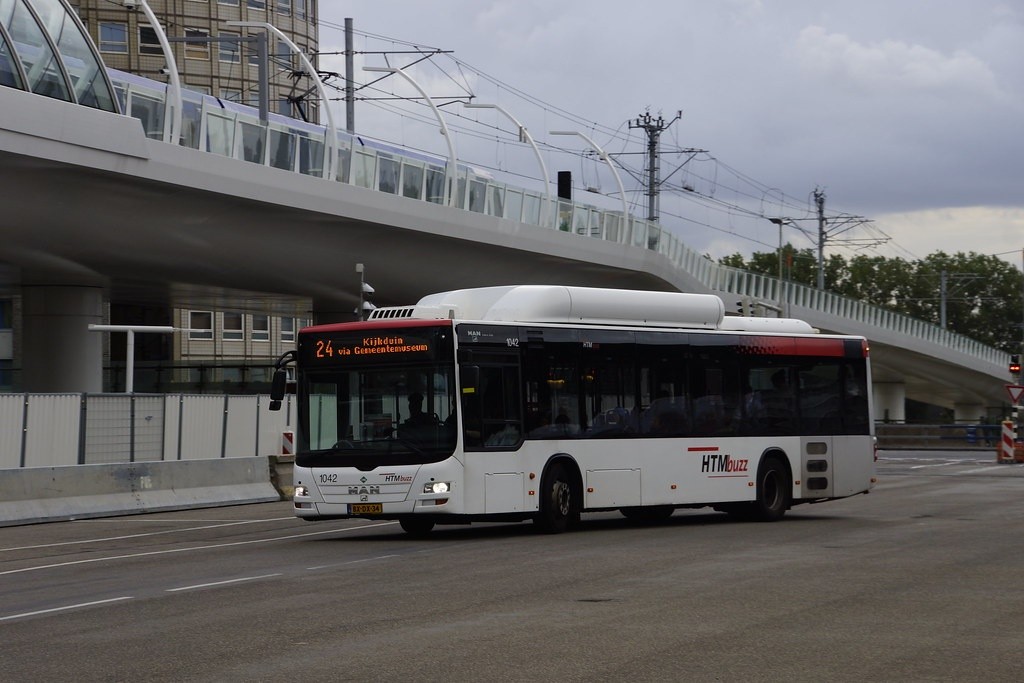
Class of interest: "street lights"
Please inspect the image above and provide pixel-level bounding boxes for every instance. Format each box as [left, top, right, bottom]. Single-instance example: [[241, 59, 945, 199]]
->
[[768, 217, 791, 306]]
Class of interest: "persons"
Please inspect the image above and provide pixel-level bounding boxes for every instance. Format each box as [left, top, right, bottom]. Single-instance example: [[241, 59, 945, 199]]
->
[[403, 394, 435, 425], [443, 395, 482, 448]]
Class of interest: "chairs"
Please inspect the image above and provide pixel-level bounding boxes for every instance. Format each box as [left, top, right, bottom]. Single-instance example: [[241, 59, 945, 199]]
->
[[589, 395, 793, 436]]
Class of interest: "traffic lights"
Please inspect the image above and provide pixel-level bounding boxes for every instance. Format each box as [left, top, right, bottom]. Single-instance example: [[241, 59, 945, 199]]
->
[[1008, 355, 1020, 373]]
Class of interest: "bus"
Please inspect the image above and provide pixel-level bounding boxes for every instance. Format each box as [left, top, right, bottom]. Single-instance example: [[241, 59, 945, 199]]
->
[[267, 285, 878, 536]]
